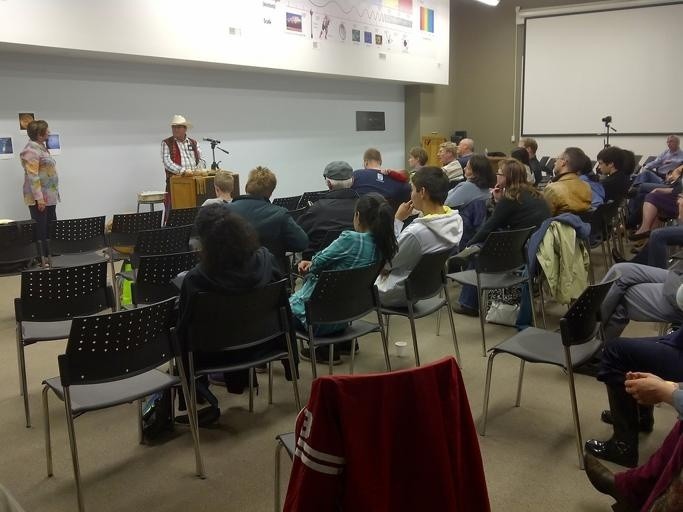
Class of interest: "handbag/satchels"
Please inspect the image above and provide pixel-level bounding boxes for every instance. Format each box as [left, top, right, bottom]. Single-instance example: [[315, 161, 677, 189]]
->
[[486, 300, 521, 327], [141, 366, 177, 443]]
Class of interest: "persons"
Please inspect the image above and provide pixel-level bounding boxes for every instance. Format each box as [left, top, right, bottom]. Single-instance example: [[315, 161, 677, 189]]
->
[[160, 114, 206, 190], [19, 119, 61, 255], [172, 134, 682, 510]]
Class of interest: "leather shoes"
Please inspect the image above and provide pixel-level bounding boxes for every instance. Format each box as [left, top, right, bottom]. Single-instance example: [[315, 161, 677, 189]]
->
[[629, 231, 650, 240], [612, 248, 626, 262], [602, 409, 654, 433], [585, 439, 638, 467], [585, 356, 602, 369]]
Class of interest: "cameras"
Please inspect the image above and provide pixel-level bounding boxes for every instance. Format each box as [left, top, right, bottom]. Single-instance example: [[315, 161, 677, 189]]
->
[[601, 115, 613, 123]]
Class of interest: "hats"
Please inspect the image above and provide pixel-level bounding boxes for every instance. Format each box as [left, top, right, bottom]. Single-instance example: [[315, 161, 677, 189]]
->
[[323, 160, 354, 180], [170, 115, 191, 128]]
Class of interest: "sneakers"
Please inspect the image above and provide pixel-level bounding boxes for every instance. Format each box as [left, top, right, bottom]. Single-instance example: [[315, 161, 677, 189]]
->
[[339, 339, 360, 354], [628, 182, 638, 196], [208, 372, 227, 386], [301, 347, 341, 365], [453, 301, 479, 317]]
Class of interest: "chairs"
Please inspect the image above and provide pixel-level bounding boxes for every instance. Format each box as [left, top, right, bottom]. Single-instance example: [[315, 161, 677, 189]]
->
[[274, 353, 462, 510], [535, 155, 682, 338], [436, 223, 538, 356], [166, 276, 301, 432], [479, 272, 624, 470], [40, 293, 207, 510], [377, 244, 460, 369], [295, 256, 392, 381], [3, 186, 332, 430]]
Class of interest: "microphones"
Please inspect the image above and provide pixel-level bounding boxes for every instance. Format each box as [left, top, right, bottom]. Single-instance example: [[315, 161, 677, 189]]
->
[[202, 137, 222, 145]]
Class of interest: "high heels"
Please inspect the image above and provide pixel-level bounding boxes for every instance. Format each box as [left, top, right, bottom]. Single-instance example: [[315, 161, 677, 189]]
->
[[584, 454, 636, 512]]
